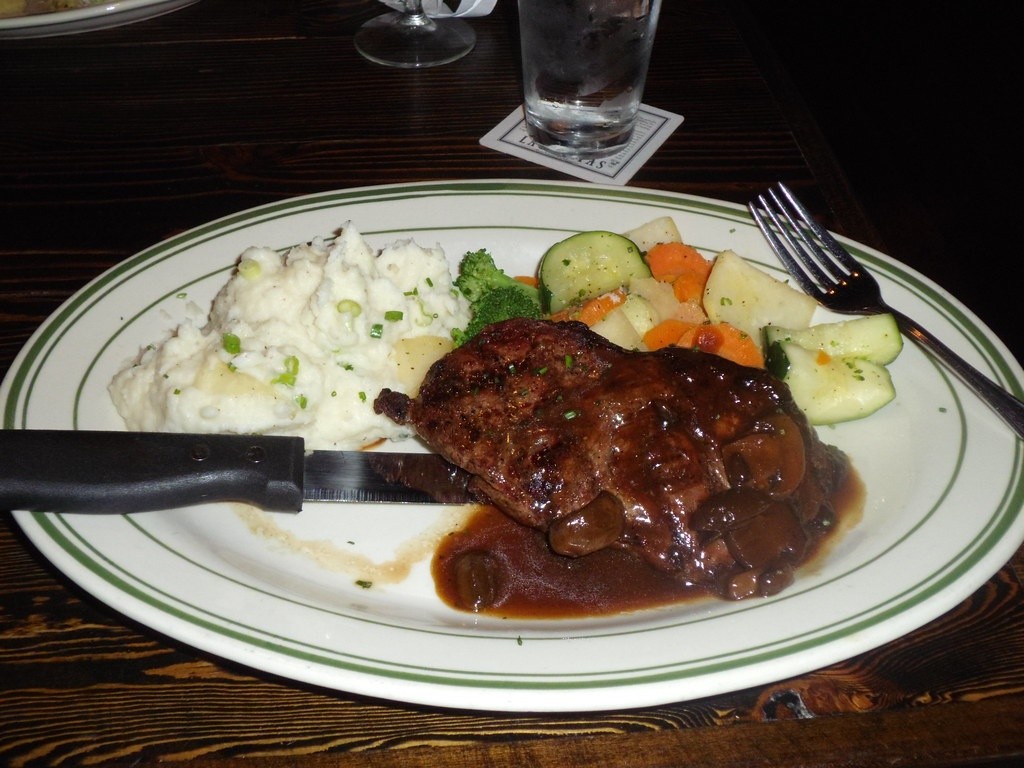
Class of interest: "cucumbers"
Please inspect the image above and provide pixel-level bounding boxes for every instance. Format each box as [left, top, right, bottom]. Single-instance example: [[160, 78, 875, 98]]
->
[[540, 218, 900, 426]]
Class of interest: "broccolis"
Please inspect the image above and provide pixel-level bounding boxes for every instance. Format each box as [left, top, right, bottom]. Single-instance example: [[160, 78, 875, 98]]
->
[[455, 252, 540, 337]]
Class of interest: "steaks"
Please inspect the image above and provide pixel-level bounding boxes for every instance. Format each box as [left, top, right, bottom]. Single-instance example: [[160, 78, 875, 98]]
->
[[375, 318, 817, 598]]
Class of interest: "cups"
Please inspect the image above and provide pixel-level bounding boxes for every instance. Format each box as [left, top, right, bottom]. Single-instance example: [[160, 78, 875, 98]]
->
[[515, 0, 664, 159]]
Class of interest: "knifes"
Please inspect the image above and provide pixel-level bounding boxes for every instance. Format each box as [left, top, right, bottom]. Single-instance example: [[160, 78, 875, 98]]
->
[[1, 429, 478, 513]]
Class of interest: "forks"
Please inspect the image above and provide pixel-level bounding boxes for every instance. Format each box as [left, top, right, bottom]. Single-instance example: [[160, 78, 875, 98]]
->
[[744, 179, 1024, 444]]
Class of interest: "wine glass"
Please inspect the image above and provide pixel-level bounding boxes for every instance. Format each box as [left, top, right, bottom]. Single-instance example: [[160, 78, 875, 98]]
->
[[353, 1, 477, 68]]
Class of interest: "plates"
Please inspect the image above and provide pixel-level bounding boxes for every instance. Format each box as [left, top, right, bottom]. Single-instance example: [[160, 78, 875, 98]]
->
[[0, 177, 1024, 715], [0, 0, 201, 40]]
